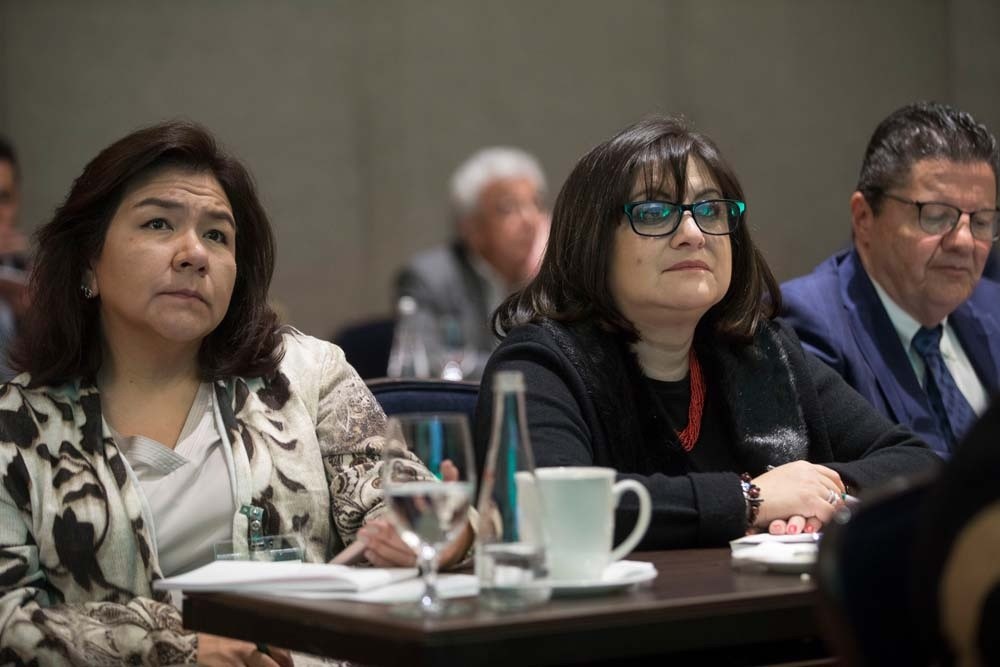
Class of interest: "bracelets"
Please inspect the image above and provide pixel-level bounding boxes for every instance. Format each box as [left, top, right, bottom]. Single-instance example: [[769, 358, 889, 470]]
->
[[740, 471, 761, 526], [447, 543, 474, 570]]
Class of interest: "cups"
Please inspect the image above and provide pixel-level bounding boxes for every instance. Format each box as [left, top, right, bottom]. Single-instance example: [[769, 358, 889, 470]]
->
[[509, 467, 653, 582]]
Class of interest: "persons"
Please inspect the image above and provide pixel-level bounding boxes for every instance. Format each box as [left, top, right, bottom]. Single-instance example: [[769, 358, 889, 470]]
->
[[762, 101, 1000, 458], [395, 146, 551, 380], [0, 121, 481, 667], [816, 393, 1000, 667], [472, 117, 954, 551], [0, 132, 40, 377]]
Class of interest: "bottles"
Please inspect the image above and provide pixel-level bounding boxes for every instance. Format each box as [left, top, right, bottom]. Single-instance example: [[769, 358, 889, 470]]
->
[[470, 370, 554, 611]]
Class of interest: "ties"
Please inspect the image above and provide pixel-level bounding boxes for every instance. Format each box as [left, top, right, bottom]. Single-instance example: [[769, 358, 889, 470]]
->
[[912, 323, 980, 454]]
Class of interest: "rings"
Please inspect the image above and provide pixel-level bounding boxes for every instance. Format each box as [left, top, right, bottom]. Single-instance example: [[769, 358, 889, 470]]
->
[[828, 486, 847, 508]]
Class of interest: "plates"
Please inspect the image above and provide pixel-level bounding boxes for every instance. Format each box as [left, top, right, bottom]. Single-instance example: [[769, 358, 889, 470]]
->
[[549, 559, 660, 598]]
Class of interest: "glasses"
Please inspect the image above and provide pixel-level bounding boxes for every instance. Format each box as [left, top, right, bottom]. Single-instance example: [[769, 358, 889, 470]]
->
[[882, 194, 1000, 242], [623, 196, 745, 237]]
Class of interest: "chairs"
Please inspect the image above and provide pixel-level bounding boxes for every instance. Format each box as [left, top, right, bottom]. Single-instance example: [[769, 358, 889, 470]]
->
[[333, 318, 484, 475]]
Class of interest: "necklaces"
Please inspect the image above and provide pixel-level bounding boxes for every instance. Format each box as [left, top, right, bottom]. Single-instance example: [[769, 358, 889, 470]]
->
[[662, 351, 705, 451]]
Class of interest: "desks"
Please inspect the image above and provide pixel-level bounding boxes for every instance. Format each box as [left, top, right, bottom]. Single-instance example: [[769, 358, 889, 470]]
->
[[182, 542, 817, 667]]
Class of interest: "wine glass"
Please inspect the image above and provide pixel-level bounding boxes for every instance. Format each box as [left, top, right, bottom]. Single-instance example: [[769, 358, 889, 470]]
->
[[380, 412, 474, 619]]
